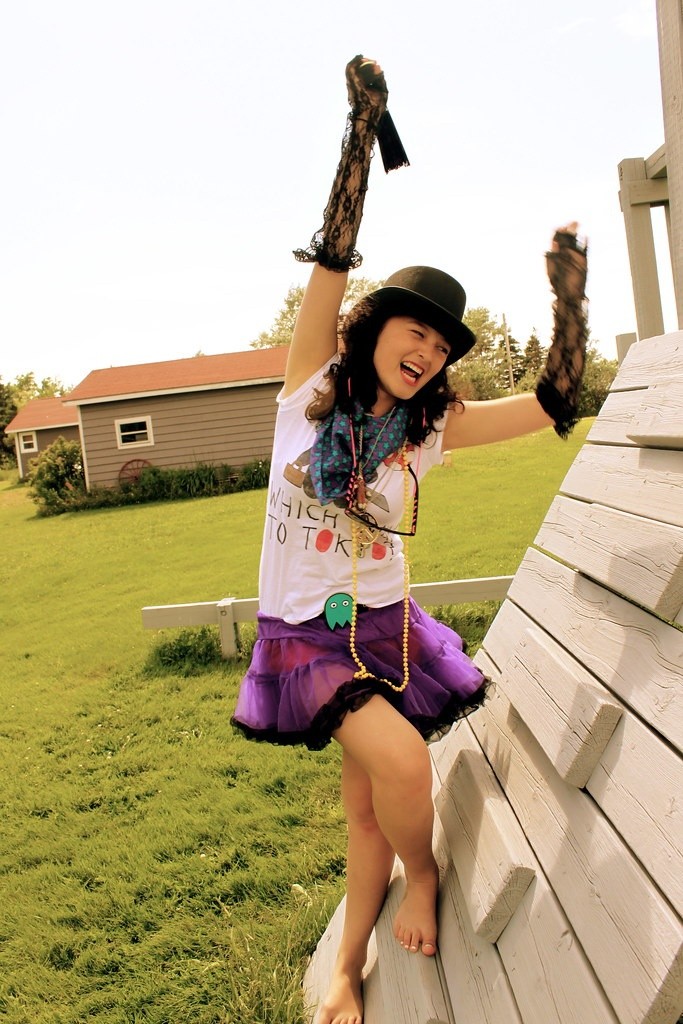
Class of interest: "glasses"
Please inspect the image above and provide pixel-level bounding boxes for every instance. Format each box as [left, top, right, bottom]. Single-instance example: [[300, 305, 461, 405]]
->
[[344, 461, 421, 537]]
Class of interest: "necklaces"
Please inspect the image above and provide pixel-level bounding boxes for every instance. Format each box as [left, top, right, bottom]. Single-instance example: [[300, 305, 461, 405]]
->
[[349, 423, 410, 692], [356, 405, 396, 510]]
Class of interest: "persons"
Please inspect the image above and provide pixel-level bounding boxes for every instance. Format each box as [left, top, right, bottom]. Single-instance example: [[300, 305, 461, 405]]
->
[[231, 53, 591, 1024]]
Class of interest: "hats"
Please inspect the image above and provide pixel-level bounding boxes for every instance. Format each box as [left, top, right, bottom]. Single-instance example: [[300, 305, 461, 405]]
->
[[342, 265, 477, 370]]
